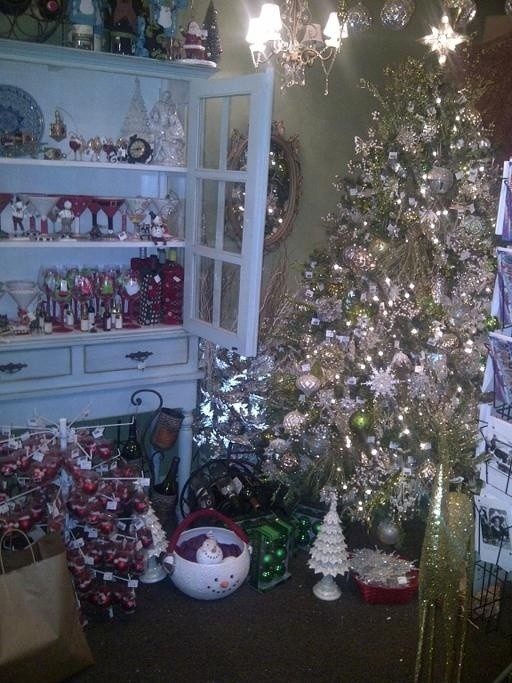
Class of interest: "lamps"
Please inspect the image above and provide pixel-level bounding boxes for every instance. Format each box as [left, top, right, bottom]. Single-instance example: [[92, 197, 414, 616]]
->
[[245, 0, 349, 96], [415, 0, 470, 66]]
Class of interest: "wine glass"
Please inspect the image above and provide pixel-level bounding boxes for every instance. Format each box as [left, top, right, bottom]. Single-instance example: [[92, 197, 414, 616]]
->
[[116, 136, 129, 166], [70, 136, 82, 162], [120, 204, 132, 240], [87, 138, 96, 163], [125, 198, 151, 241], [79, 137, 87, 162], [110, 215, 112, 216], [104, 136, 116, 164], [58, 194, 94, 241], [94, 136, 103, 163], [1, 192, 13, 241], [6, 281, 40, 334], [38, 265, 142, 333], [150, 198, 182, 242], [87, 193, 104, 242], [16, 193, 61, 242]]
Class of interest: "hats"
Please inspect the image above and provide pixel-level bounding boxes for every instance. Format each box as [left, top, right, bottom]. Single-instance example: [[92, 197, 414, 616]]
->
[[149, 208, 157, 220], [491, 510, 506, 522], [13, 195, 22, 204]]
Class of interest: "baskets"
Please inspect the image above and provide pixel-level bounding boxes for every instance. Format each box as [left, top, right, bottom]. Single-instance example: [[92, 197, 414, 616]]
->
[[353, 572, 418, 605]]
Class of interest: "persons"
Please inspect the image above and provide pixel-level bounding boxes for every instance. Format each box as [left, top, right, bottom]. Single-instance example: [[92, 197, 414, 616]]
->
[[147, 210, 167, 250], [57, 200, 77, 240], [9, 196, 32, 239], [151, 89, 186, 167], [179, 21, 206, 59]]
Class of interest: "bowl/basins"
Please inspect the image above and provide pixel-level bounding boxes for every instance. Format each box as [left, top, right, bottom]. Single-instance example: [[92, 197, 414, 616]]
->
[[349, 553, 420, 605], [165, 528, 256, 603]]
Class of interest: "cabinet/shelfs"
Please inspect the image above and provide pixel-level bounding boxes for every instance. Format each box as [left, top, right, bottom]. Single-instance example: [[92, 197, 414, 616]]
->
[[471, 158, 512, 571]]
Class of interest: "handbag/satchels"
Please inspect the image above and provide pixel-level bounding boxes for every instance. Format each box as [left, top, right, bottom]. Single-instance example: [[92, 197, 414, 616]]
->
[[1, 527, 95, 682]]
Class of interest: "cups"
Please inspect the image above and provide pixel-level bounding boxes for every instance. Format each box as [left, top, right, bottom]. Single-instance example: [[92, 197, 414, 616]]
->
[[96, 196, 123, 241]]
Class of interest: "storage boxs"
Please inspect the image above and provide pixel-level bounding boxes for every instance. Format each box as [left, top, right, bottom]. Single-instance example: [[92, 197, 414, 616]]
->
[[0, 38, 274, 523]]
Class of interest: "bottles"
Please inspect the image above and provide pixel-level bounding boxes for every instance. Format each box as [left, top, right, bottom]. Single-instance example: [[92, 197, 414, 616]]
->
[[103, 305, 111, 331], [159, 249, 166, 266], [63, 304, 74, 326], [208, 463, 243, 508], [115, 304, 123, 328], [38, 304, 45, 328], [123, 418, 142, 459], [160, 457, 180, 495], [88, 300, 95, 325], [44, 311, 52, 334], [230, 467, 262, 509], [191, 474, 215, 509], [161, 248, 184, 325], [111, 303, 117, 325], [140, 247, 147, 260], [80, 303, 88, 332]]
